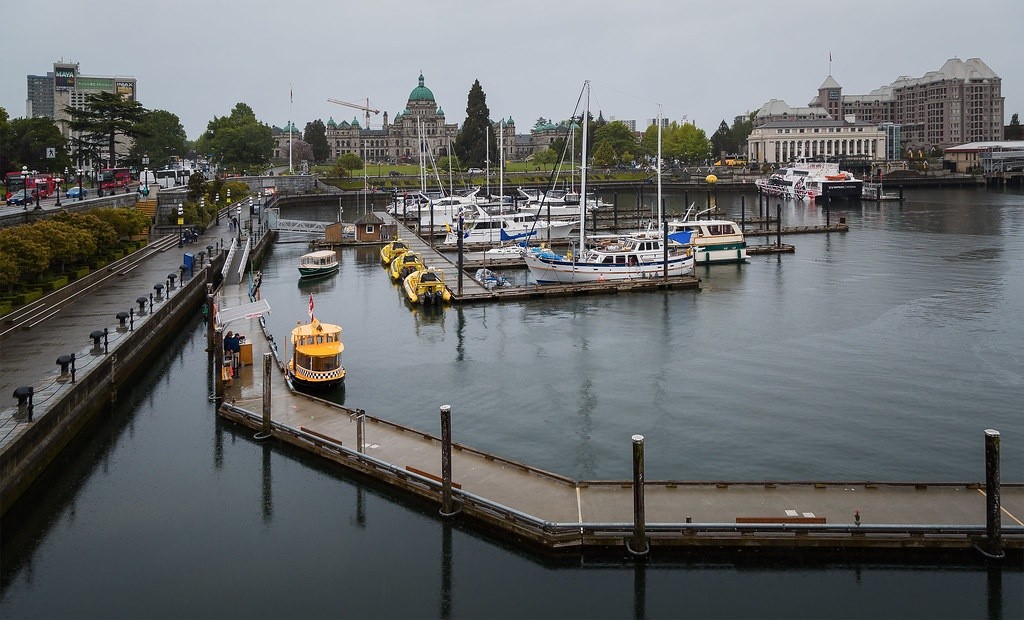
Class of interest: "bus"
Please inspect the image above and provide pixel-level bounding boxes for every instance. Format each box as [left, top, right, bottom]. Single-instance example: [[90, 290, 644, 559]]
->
[[140, 169, 197, 190], [3, 171, 54, 200], [97, 168, 131, 189], [714, 155, 747, 166]]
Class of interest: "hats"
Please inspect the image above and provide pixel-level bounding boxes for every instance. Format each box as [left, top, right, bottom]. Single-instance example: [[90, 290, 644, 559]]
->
[[186, 228, 189, 230]]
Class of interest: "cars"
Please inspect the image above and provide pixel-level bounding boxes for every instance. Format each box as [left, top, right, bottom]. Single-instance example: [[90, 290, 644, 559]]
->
[[6, 186, 87, 206], [389, 171, 403, 176], [468, 168, 483, 173]]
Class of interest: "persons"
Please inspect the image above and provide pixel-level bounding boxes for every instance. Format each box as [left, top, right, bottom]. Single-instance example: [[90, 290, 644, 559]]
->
[[183, 228, 191, 244], [223, 330, 240, 378], [193, 232, 199, 243], [228, 217, 238, 231], [127, 184, 130, 193], [110, 188, 115, 195], [139, 181, 150, 199], [98, 187, 103, 197]]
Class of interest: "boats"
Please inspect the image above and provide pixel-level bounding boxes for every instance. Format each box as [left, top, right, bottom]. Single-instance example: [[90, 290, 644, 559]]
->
[[768, 154, 840, 198], [298, 250, 340, 281], [380, 236, 411, 265], [390, 250, 451, 304], [288, 292, 346, 394], [474, 268, 512, 291], [628, 201, 751, 264]]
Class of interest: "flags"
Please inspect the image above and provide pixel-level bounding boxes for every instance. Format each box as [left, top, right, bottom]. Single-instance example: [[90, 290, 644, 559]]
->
[[307, 292, 315, 319]]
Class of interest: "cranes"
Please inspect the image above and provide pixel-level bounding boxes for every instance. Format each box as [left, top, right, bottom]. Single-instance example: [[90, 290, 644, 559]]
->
[[326, 98, 380, 128]]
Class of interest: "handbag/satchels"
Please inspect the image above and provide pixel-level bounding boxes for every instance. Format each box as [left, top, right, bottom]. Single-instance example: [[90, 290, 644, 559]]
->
[[227, 223, 229, 227]]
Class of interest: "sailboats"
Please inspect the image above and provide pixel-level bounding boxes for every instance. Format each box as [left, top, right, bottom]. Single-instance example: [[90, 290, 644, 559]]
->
[[387, 80, 699, 285]]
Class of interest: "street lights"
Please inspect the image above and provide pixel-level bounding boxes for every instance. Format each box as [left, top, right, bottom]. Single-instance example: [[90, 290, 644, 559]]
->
[[34, 178, 62, 209], [64, 167, 94, 200], [144, 167, 148, 189], [21, 166, 29, 209]]
